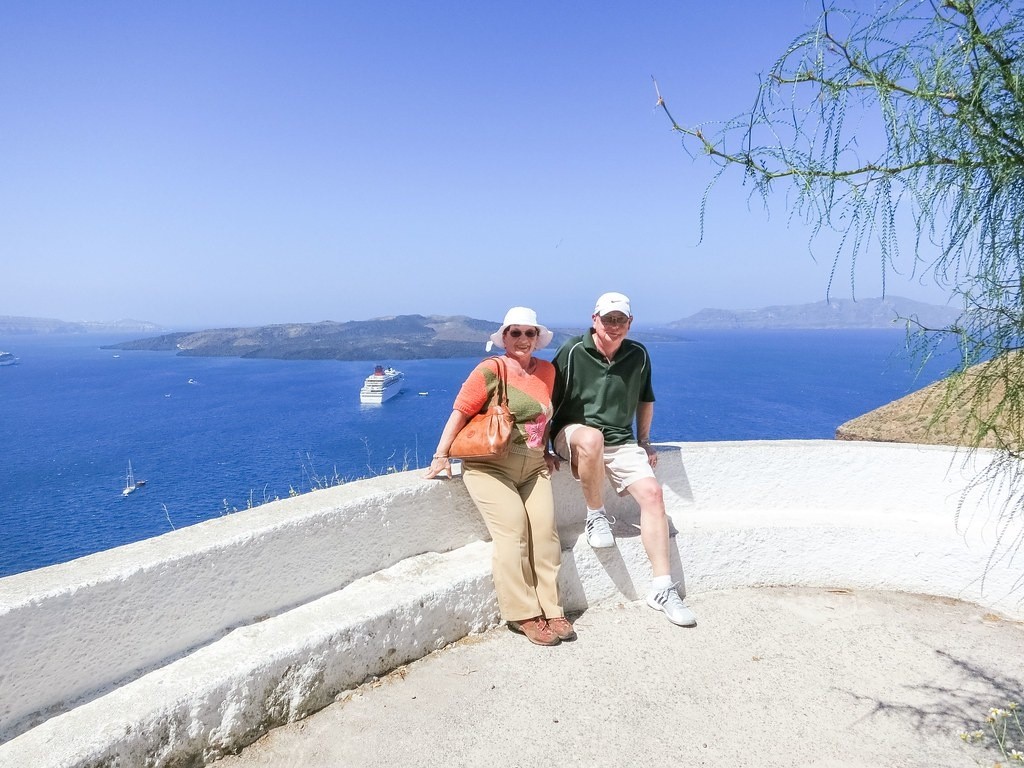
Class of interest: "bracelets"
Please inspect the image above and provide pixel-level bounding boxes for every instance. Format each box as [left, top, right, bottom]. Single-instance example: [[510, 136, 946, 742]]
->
[[638, 438, 651, 446], [432, 454, 448, 459]]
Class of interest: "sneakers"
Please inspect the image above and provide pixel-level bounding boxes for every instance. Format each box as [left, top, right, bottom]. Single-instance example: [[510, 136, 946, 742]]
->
[[648, 581, 695, 625], [545, 617, 575, 639], [584, 511, 616, 548], [510, 616, 559, 645]]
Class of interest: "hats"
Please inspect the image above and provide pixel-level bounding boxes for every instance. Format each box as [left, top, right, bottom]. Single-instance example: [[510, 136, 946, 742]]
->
[[491, 307, 553, 349], [594, 292, 630, 319]]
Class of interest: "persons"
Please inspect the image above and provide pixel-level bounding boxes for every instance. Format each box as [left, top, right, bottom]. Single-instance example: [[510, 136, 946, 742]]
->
[[418, 307, 575, 645], [550, 293, 696, 627]]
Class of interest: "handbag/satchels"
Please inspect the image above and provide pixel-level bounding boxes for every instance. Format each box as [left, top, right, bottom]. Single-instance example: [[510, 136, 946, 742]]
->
[[447, 356, 514, 462]]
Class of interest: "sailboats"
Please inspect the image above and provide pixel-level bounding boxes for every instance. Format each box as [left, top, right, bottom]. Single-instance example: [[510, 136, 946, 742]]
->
[[123, 460, 137, 496]]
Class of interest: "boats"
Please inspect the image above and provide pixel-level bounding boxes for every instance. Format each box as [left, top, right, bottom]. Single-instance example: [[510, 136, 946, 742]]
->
[[189, 379, 198, 384], [359, 364, 405, 403], [137, 481, 146, 486], [0, 352, 19, 366], [114, 353, 120, 358], [419, 392, 429, 396]]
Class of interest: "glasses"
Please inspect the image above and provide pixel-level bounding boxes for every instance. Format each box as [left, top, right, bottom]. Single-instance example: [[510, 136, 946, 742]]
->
[[507, 328, 538, 338], [598, 313, 629, 325]]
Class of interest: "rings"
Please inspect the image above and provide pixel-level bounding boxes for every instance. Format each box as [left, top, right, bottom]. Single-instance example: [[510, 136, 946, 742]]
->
[[654, 461, 656, 462]]
